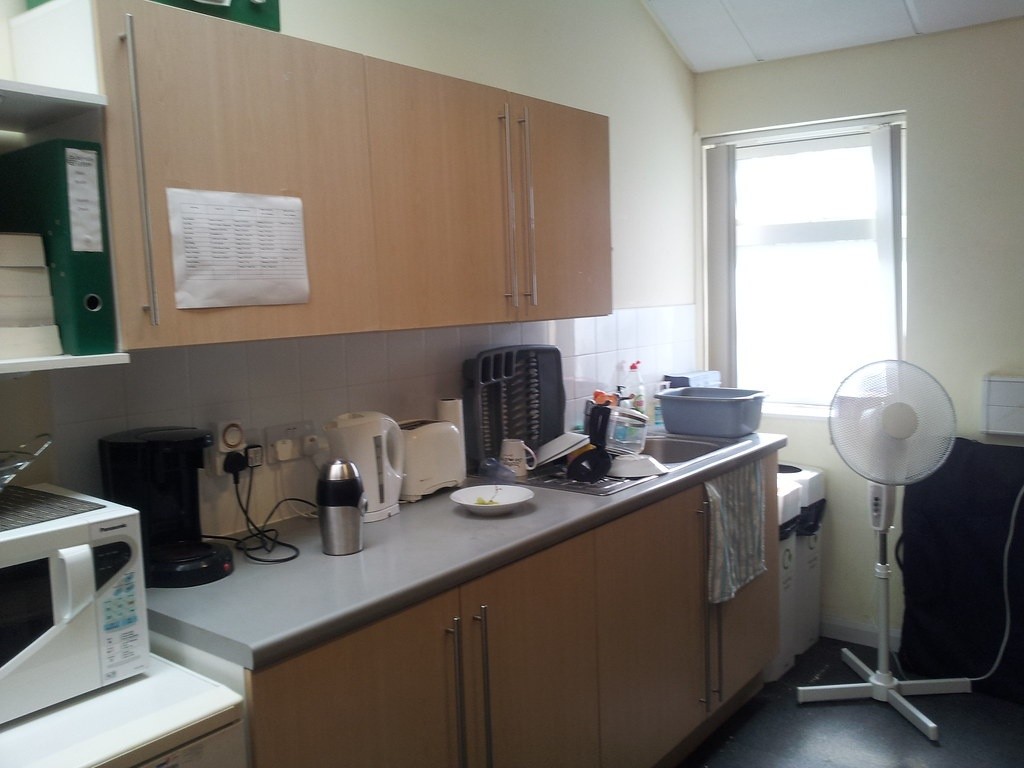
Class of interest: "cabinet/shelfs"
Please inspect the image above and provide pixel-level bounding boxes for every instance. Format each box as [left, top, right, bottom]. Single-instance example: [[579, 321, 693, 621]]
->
[[0, 0, 613, 377], [147, 420, 790, 768]]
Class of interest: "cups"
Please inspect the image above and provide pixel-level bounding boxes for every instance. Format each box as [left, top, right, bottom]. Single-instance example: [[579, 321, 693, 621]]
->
[[498, 439, 536, 477]]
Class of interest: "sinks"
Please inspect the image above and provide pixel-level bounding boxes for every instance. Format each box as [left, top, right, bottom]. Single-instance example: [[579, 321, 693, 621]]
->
[[637, 435, 721, 464]]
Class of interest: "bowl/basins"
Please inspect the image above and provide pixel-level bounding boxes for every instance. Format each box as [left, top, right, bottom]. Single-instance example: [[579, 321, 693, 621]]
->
[[655, 387, 767, 438], [586, 406, 647, 455], [567, 447, 612, 484]]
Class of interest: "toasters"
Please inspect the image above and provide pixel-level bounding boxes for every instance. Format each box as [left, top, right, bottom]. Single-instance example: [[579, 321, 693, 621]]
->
[[395, 419, 465, 502]]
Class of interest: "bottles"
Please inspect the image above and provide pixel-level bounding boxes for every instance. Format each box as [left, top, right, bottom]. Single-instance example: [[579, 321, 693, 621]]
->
[[627, 361, 644, 412]]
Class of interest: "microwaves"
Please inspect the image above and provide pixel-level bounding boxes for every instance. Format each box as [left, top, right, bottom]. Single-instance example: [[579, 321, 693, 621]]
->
[[0, 483, 149, 724]]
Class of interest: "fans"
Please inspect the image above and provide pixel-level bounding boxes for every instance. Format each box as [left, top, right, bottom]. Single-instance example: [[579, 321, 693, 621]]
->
[[798, 357, 974, 742]]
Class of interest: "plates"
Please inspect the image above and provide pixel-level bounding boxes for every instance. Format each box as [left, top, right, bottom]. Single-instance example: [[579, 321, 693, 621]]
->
[[450, 485, 534, 516], [605, 454, 667, 477], [536, 433, 590, 466]]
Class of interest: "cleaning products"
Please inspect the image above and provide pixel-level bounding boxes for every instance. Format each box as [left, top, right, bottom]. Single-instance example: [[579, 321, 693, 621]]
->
[[632, 383, 647, 414]]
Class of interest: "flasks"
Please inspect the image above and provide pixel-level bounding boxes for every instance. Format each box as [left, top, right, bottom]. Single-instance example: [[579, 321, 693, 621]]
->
[[318, 461, 367, 556]]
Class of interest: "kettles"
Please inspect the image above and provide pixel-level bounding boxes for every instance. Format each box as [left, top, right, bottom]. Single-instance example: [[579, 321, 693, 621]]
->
[[325, 412, 403, 523]]
